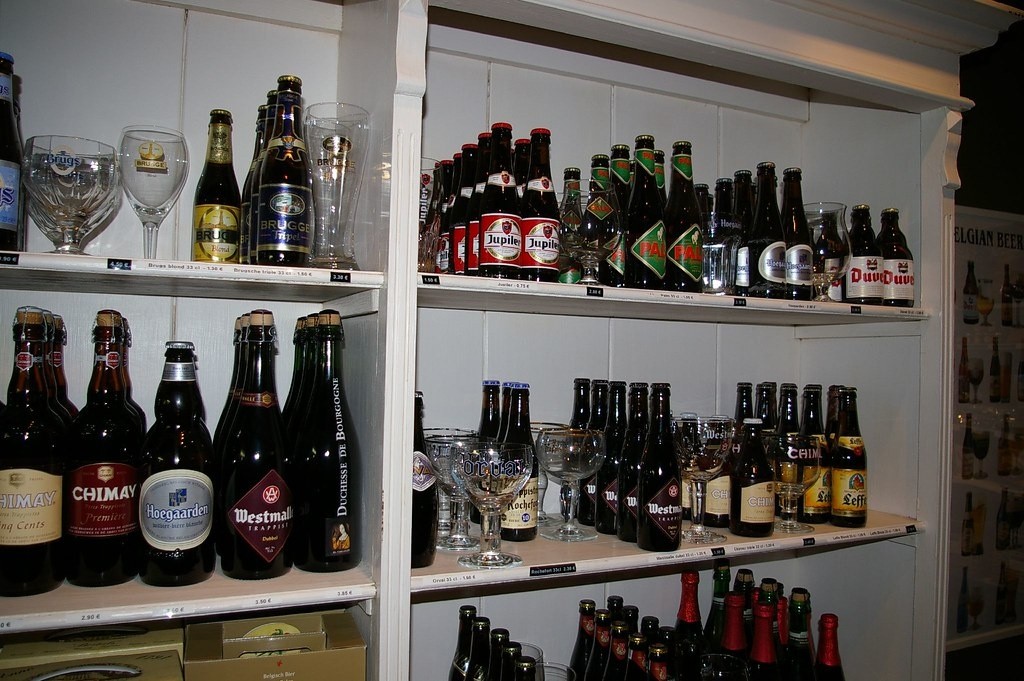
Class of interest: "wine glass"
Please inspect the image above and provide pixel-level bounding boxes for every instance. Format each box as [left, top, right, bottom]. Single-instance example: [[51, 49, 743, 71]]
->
[[113, 125, 191, 260], [423, 427, 481, 552], [535, 426, 607, 542], [529, 420, 571, 528], [448, 443, 533, 570], [764, 436, 820, 534], [802, 201, 852, 305], [557, 177, 622, 287], [671, 417, 735, 547], [20, 133, 124, 256]]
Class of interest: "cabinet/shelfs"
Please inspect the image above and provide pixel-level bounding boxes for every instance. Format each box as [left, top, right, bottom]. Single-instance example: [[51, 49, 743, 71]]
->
[[0, 0, 1024, 681]]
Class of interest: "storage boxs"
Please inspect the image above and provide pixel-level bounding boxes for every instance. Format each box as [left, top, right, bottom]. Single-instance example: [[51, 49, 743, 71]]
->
[[0, 618, 184, 668], [0, 651, 181, 681], [181, 611, 368, 681]]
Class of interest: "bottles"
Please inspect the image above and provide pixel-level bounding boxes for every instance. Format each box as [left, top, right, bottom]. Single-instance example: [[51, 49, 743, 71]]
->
[[0, 50, 915, 681]]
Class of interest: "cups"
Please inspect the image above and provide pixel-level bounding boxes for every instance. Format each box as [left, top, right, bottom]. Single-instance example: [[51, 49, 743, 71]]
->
[[531, 662, 577, 681], [301, 99, 372, 271], [510, 640, 546, 681], [698, 236, 736, 298], [417, 155, 442, 274]]
